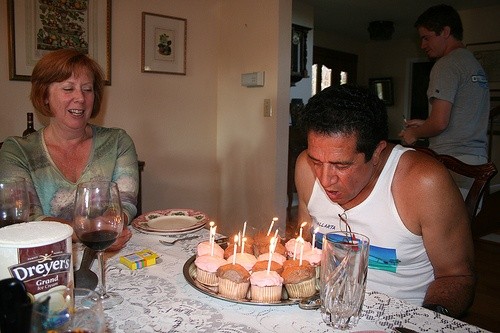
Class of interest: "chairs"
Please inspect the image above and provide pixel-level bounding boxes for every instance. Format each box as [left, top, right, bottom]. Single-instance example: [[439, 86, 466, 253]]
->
[[404, 146, 498, 223]]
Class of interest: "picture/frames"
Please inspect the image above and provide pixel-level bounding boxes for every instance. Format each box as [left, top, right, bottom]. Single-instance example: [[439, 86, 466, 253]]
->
[[465, 41, 500, 136], [7, 0, 112, 86], [142, 12, 186, 76]]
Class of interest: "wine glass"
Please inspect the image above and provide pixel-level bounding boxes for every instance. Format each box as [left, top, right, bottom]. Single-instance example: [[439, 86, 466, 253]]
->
[[73, 180, 124, 310]]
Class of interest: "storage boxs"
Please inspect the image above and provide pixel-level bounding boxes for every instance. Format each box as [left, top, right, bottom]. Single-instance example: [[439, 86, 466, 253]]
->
[[119, 249, 160, 270]]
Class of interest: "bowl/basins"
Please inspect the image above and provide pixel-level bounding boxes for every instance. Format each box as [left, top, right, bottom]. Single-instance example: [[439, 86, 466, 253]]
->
[[106, 229, 132, 252]]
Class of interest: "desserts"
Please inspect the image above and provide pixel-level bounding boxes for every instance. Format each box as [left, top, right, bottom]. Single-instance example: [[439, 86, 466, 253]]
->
[[193, 231, 323, 301]]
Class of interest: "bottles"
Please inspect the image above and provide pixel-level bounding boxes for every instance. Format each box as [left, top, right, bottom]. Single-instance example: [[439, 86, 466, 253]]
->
[[290, 97, 304, 126], [22, 113, 36, 136]]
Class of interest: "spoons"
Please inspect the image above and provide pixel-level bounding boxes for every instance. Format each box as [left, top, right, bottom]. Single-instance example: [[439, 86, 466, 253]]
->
[[159, 236, 199, 246]]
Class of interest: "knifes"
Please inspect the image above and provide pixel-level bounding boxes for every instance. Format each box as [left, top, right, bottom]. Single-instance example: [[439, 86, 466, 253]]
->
[[394, 327, 418, 333]]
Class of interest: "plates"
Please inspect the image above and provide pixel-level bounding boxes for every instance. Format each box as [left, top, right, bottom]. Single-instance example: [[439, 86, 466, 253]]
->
[[131, 207, 208, 235]]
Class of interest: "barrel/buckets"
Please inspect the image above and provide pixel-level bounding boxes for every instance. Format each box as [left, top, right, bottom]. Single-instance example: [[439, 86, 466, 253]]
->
[[0, 220, 73, 294]]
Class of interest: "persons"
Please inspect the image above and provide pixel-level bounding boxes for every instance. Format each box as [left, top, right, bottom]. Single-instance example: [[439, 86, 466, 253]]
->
[[0, 46, 139, 253], [398, 5, 490, 165], [294, 84, 483, 323]]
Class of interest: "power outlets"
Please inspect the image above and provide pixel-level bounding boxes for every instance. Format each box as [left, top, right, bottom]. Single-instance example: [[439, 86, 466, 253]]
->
[[264, 99, 272, 117]]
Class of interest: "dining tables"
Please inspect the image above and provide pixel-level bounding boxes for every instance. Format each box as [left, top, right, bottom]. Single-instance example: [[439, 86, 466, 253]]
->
[[72, 225, 492, 333]]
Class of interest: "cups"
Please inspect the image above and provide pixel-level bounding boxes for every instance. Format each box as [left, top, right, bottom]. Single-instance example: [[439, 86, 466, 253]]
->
[[319, 231, 370, 331], [30, 287, 105, 333], [0, 176, 29, 228]]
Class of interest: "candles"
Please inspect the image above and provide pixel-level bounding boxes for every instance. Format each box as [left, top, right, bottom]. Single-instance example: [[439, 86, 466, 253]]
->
[[209, 218, 321, 273]]
